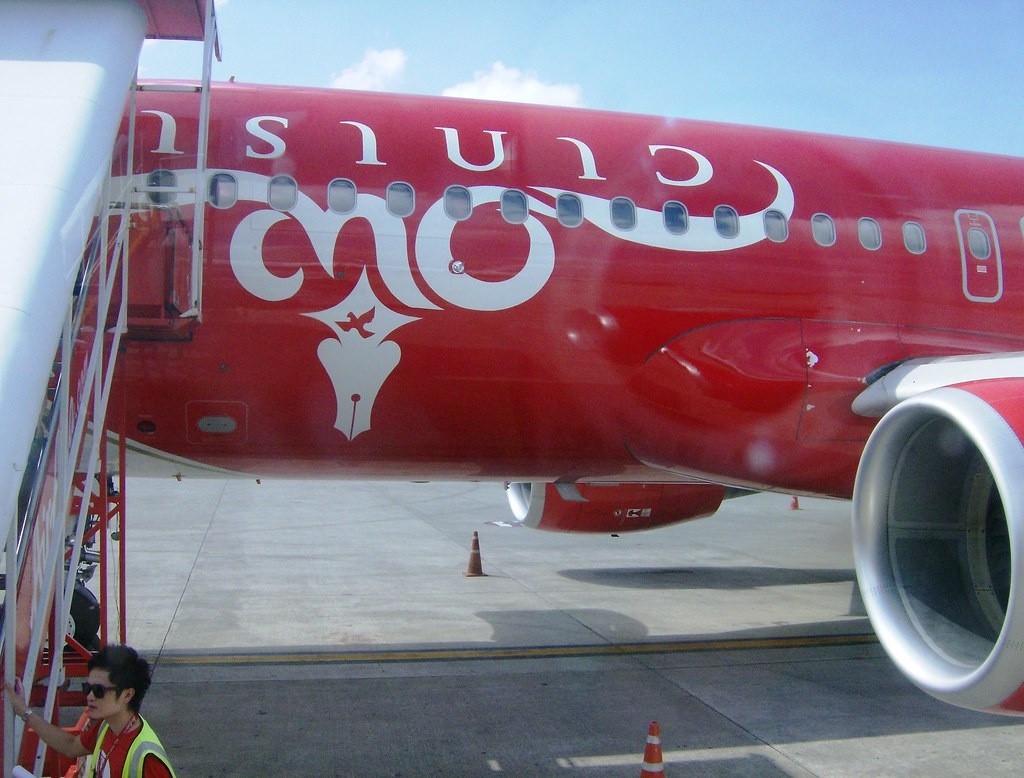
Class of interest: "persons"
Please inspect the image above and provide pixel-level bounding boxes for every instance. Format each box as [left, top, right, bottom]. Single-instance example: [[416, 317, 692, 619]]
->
[[0, 645, 176, 778]]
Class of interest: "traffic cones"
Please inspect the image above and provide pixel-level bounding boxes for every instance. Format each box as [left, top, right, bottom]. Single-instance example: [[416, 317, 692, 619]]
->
[[640, 721, 668, 778], [462, 531, 488, 577], [789, 496, 799, 510]]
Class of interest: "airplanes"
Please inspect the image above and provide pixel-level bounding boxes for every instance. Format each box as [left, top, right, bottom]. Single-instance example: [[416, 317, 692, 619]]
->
[[0, 0, 1024, 778]]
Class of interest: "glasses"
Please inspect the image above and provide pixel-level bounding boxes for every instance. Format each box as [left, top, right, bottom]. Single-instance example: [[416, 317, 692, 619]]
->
[[82, 681, 118, 699]]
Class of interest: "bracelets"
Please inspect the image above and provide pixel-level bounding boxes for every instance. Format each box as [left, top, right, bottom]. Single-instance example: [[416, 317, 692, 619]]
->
[[22, 708, 33, 722]]
